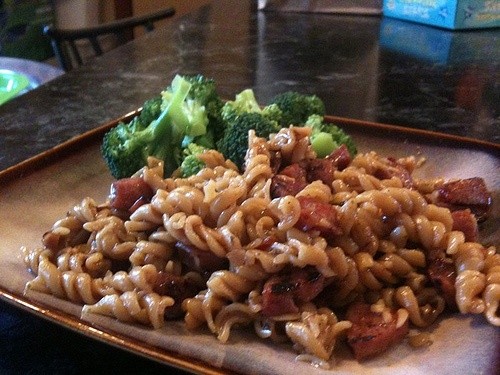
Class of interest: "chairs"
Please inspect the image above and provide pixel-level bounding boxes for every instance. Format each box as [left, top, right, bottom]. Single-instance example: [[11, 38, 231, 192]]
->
[[42, 7, 176, 75]]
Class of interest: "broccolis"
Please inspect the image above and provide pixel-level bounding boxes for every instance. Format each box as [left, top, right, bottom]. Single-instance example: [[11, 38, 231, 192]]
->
[[96, 70, 363, 179]]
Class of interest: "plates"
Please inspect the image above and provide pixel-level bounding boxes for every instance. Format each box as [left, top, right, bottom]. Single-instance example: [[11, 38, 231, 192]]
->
[[1, 108, 499, 375]]
